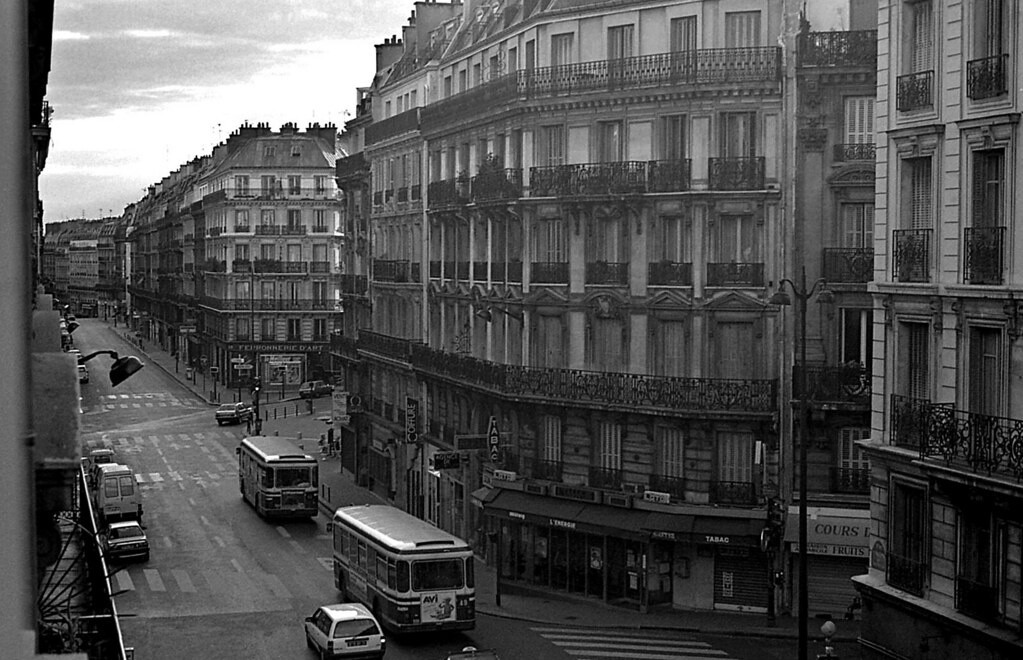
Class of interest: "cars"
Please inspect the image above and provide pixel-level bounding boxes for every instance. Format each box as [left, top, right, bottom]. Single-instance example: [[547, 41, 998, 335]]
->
[[87, 448, 118, 482], [59, 317, 88, 383], [102, 521, 150, 567], [304, 603, 387, 660]]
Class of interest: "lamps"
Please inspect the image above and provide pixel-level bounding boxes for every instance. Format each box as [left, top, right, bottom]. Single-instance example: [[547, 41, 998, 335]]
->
[[475, 306, 524, 328], [53, 298, 145, 387]]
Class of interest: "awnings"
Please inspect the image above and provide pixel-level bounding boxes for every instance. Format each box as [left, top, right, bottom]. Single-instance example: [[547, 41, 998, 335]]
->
[[784, 506, 870, 546], [483, 488, 766, 544]]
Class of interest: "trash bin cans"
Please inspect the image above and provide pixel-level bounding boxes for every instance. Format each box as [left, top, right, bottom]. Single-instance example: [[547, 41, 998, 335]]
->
[[186, 368, 192, 380], [305, 399, 312, 411], [255, 419, 262, 431], [139, 339, 142, 346]]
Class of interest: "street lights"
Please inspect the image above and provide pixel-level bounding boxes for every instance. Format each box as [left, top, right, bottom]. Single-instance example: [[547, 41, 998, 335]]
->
[[770, 265, 834, 660]]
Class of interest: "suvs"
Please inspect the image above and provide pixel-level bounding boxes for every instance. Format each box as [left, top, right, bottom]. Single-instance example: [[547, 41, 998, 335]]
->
[[298, 380, 331, 398], [215, 402, 252, 426]]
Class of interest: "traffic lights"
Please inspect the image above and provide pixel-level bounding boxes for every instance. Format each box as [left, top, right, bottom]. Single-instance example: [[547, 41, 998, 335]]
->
[[775, 568, 785, 585], [255, 383, 261, 393], [765, 494, 786, 548]]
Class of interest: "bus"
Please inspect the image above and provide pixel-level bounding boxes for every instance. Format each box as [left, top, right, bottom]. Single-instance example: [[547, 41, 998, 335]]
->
[[236, 436, 318, 520], [331, 505, 476, 640]]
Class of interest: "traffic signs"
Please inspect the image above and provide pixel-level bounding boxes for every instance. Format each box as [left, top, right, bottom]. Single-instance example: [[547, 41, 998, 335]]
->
[[234, 365, 253, 369]]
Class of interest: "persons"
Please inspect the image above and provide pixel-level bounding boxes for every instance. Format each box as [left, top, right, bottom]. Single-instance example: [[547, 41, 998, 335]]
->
[[252, 379, 261, 405], [845, 597, 860, 621]]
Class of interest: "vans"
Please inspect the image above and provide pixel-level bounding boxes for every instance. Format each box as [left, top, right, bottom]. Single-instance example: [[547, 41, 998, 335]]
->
[[96, 465, 141, 523]]
